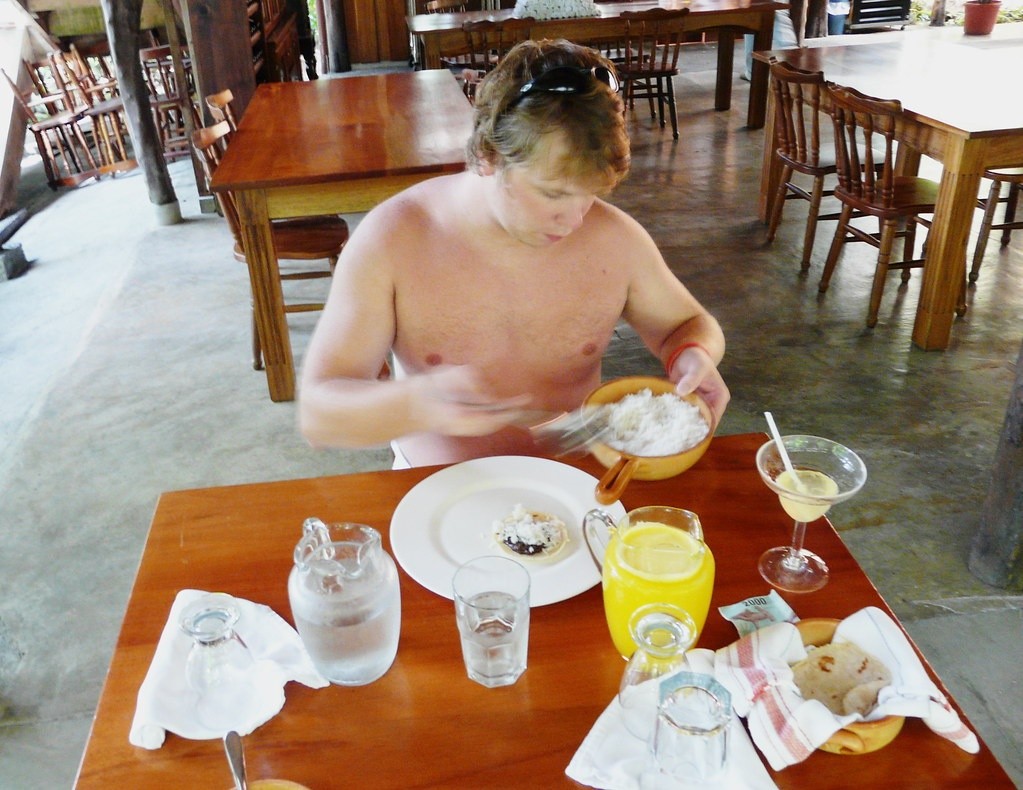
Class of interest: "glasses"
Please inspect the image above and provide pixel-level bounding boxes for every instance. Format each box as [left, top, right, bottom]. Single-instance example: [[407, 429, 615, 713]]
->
[[496, 65, 621, 122]]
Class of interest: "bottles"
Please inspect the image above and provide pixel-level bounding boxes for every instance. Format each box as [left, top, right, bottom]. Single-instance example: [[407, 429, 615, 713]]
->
[[288, 517, 403, 686]]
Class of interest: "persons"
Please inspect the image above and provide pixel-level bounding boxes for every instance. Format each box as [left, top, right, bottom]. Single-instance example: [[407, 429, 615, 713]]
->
[[298, 38, 731, 470], [826, 0, 850, 35], [740, 0, 798, 83]]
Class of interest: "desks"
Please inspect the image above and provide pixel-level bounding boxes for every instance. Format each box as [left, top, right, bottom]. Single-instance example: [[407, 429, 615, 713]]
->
[[751, 21, 1023, 353], [72, 435, 1022, 790], [404, 0, 792, 127], [209, 68, 476, 402]]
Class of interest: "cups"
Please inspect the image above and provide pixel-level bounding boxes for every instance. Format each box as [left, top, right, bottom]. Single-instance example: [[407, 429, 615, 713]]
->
[[452, 557, 531, 687], [650, 672, 733, 776]]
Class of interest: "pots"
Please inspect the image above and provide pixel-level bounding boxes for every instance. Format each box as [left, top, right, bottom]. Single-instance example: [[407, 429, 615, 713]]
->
[[584, 376, 715, 505]]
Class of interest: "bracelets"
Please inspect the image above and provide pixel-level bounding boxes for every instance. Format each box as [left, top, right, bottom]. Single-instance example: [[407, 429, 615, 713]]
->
[[665, 341, 709, 375]]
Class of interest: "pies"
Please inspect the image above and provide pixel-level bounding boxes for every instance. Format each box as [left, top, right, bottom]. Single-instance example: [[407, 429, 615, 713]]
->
[[495, 511, 570, 559], [791, 643, 890, 717]]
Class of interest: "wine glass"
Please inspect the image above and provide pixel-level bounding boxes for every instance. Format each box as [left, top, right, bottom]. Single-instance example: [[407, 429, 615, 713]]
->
[[622, 603, 700, 742], [180, 595, 251, 706], [755, 433, 867, 593]]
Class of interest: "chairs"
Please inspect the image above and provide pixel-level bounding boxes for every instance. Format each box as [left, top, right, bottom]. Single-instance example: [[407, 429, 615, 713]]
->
[[424, 0, 690, 141], [818, 80, 941, 329], [190, 88, 393, 382], [765, 56, 887, 274], [922, 168, 1023, 287], [848, 0, 911, 34], [0, 0, 306, 191]]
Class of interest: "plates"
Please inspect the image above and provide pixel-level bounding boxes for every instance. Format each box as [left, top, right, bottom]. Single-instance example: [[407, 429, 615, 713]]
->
[[390, 455, 630, 612]]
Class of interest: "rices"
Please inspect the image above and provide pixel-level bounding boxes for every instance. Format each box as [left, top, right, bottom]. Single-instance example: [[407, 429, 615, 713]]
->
[[600, 387, 709, 457]]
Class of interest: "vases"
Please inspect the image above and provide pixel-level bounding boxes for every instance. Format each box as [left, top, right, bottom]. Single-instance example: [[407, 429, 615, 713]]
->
[[964, 0, 1002, 35]]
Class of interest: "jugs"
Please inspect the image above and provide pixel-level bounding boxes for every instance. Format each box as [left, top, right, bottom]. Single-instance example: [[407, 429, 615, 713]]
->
[[582, 504, 715, 662]]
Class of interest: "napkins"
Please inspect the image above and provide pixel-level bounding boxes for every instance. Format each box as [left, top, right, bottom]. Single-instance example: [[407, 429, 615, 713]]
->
[[128, 589, 331, 751], [710, 605, 979, 772], [566, 648, 779, 790]]
